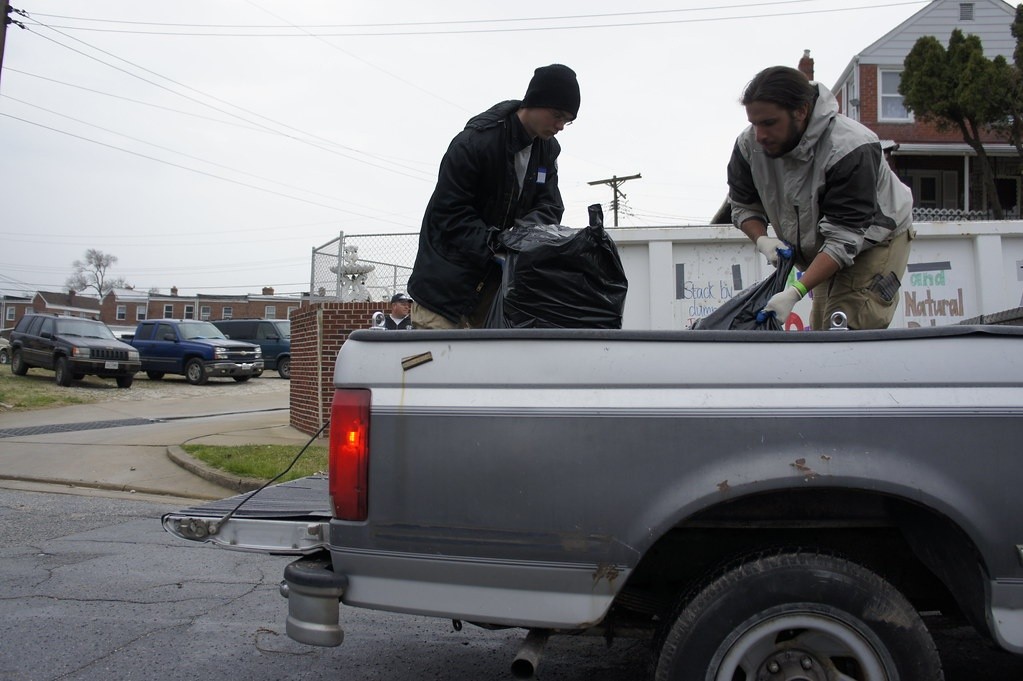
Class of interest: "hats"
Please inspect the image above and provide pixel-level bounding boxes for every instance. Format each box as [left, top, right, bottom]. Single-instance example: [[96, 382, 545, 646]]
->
[[520, 64, 580, 119], [389, 294, 414, 304]]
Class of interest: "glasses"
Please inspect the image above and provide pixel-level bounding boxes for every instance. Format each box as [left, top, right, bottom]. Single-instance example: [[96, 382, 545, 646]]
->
[[548, 109, 572, 125]]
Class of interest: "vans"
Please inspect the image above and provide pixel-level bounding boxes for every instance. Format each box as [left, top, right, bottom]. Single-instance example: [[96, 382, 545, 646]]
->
[[208, 317, 291, 379]]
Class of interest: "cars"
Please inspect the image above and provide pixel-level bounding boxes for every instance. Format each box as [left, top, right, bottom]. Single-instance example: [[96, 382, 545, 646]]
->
[[8, 312, 142, 388], [0, 335, 12, 363]]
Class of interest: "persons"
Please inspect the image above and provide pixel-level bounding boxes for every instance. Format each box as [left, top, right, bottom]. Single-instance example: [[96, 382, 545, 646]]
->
[[727, 65, 916, 331], [374, 291, 413, 330], [406, 63, 581, 330]]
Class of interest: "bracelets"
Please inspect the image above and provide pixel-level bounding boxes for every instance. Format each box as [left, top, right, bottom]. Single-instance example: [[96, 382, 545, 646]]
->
[[792, 280, 808, 298]]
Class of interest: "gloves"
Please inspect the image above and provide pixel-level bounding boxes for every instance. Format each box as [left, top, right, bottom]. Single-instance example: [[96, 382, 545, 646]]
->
[[764, 285, 802, 324], [756, 235, 788, 269]]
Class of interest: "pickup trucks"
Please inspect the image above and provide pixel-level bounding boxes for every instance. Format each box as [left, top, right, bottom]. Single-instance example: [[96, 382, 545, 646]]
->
[[115, 317, 265, 384], [161, 299, 1023, 681]]
